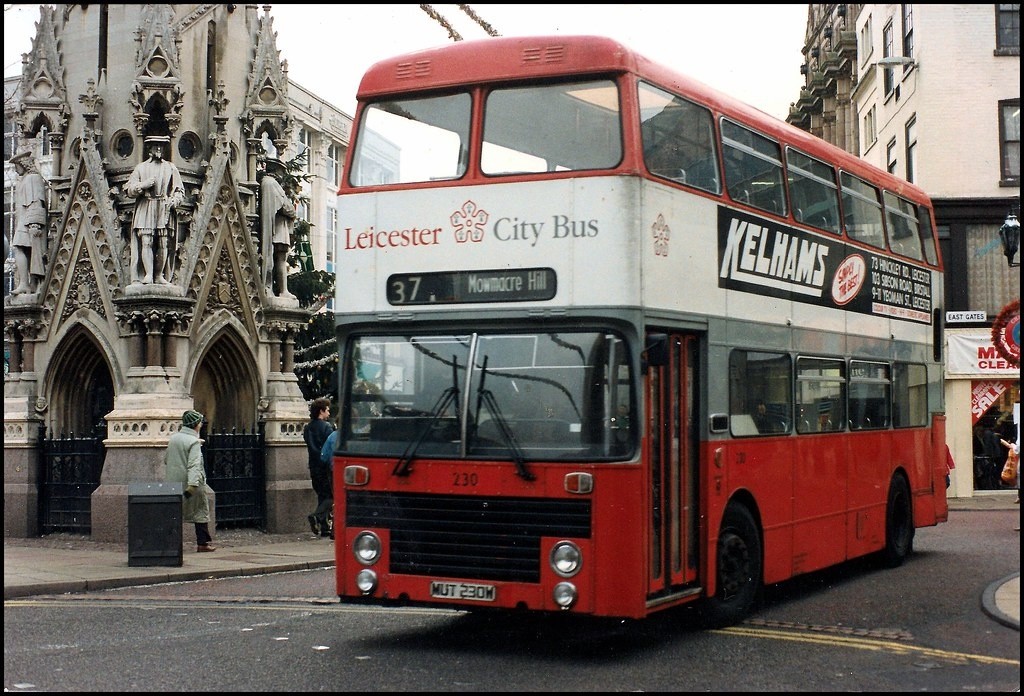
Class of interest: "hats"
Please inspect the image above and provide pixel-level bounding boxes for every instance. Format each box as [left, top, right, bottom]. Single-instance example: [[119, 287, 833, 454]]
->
[[182, 411, 203, 425]]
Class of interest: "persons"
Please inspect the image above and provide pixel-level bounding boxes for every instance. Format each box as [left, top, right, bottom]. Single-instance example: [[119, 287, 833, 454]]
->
[[304, 399, 333, 537], [163, 411, 216, 553], [321, 412, 359, 540], [1010, 423, 1020, 504], [415, 346, 465, 419], [945, 444, 955, 489], [973, 423, 1006, 489]]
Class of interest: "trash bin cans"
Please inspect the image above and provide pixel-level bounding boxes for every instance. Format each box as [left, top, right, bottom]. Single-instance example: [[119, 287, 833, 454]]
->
[[127, 480, 185, 567]]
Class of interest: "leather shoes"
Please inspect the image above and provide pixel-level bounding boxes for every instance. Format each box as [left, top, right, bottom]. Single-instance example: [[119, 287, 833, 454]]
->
[[197, 545, 216, 552]]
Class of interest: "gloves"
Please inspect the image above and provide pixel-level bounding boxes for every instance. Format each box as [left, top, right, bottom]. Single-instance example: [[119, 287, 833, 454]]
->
[[183, 486, 198, 499]]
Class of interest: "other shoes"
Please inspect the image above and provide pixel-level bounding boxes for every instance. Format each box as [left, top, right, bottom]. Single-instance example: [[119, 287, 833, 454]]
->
[[330, 529, 334, 539], [321, 528, 331, 537], [308, 513, 321, 535], [1014, 527, 1021, 531], [1014, 497, 1020, 503]]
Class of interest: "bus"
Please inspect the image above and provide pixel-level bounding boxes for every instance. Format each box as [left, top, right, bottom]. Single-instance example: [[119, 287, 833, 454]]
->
[[331, 33, 951, 627]]
